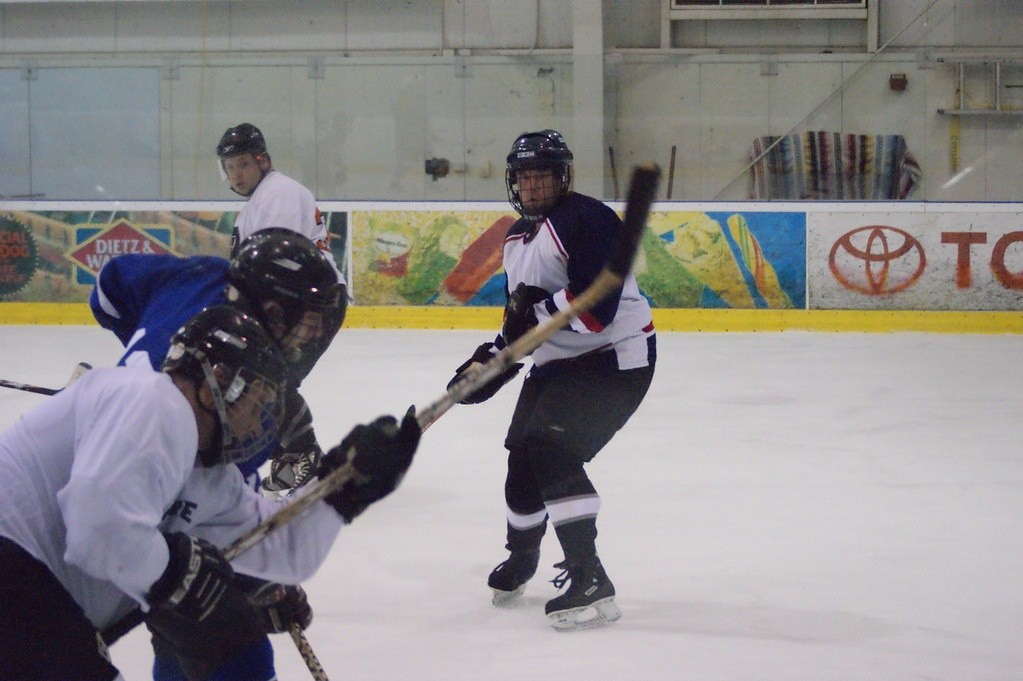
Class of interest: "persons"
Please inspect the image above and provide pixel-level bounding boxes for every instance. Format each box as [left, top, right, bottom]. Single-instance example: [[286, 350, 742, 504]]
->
[[0, 305, 314, 681], [216, 123, 349, 494], [88, 226, 423, 681], [446, 128, 658, 615]]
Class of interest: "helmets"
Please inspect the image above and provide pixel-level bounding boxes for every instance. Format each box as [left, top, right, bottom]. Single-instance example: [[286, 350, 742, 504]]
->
[[226, 228, 337, 359], [505, 129, 572, 222], [171, 305, 288, 466], [216, 123, 267, 159]]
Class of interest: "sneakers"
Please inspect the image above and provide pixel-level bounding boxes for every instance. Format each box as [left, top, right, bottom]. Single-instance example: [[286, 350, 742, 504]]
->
[[546, 553, 622, 631], [488, 544, 543, 607], [262, 458, 293, 497], [285, 448, 324, 499]]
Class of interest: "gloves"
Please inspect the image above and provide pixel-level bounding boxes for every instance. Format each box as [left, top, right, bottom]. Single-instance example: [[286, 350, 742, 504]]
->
[[141, 531, 247, 629], [247, 581, 313, 633], [502, 283, 554, 356], [315, 405, 420, 524], [446, 334, 524, 405]]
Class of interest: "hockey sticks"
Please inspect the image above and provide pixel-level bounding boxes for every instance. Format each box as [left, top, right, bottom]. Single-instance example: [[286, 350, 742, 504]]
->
[[99, 157, 666, 647], [287, 620, 330, 681], [0, 361, 93, 398]]
